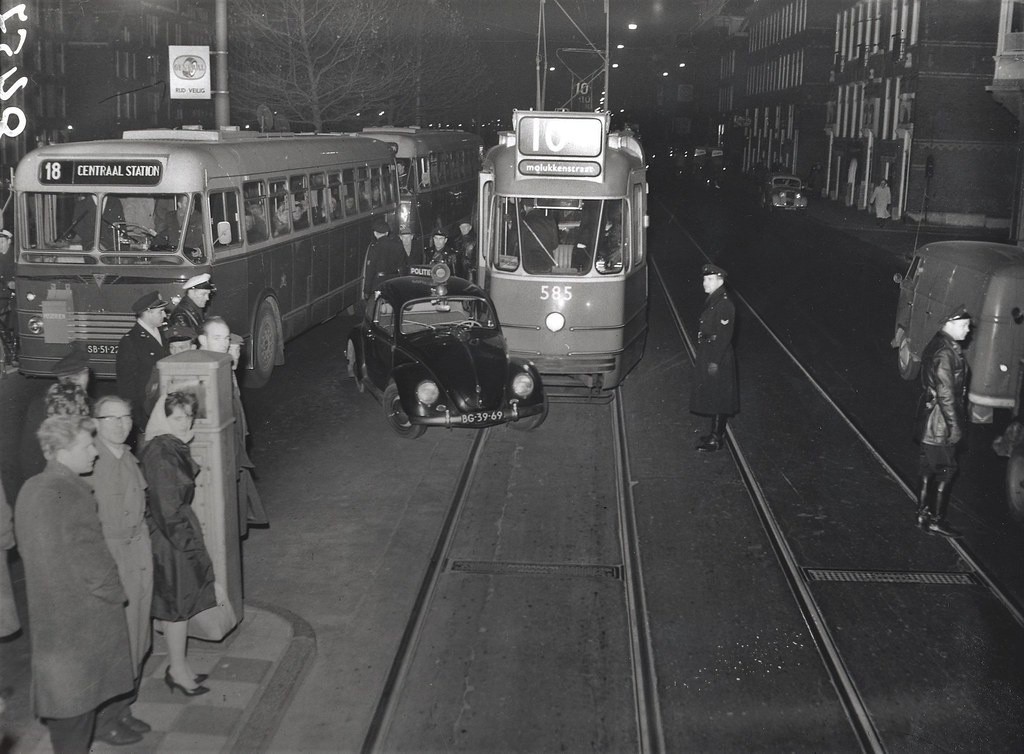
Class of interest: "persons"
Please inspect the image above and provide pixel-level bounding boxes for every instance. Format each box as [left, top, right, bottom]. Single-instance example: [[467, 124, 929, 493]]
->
[[46, 384, 90, 417], [17, 350, 88, 476], [72, 194, 129, 265], [227, 332, 269, 537], [246, 161, 410, 243], [164, 325, 198, 356], [427, 229, 458, 276], [519, 198, 559, 273], [197, 315, 231, 354], [364, 220, 404, 300], [452, 217, 477, 284], [913, 304, 969, 537], [871, 179, 893, 229], [116, 289, 171, 461], [605, 215, 621, 267], [809, 162, 827, 199], [167, 273, 215, 336], [14, 413, 136, 754], [138, 391, 219, 695], [82, 392, 155, 747], [690, 266, 740, 452], [0, 229, 21, 367], [163, 193, 206, 262]]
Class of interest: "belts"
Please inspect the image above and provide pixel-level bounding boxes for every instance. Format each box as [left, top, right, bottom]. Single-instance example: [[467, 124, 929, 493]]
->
[[697, 331, 716, 343]]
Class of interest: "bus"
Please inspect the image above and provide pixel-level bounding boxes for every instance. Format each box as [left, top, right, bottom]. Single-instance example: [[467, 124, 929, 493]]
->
[[473, 109, 651, 406], [7, 126, 486, 395]]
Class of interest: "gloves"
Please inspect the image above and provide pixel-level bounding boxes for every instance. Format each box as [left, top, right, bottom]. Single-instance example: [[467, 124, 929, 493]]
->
[[946, 423, 963, 446]]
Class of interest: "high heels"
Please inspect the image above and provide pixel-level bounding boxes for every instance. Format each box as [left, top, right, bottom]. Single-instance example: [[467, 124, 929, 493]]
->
[[165, 664, 211, 696]]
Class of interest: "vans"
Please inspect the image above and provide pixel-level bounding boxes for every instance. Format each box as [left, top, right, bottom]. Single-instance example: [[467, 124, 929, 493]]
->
[[891, 240, 1024, 438]]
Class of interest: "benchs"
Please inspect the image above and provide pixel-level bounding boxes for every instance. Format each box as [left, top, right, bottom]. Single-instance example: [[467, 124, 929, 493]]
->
[[379, 310, 468, 335]]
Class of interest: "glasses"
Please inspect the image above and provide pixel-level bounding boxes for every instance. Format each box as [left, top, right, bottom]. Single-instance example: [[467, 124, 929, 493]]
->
[[95, 414, 133, 423]]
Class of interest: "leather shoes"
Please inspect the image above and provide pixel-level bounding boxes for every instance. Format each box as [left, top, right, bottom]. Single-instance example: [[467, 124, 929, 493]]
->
[[92, 714, 151, 746]]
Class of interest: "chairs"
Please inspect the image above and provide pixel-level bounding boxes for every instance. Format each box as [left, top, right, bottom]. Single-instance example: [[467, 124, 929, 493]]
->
[[118, 190, 370, 258]]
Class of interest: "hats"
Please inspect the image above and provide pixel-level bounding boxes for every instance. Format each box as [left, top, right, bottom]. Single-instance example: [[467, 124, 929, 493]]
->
[[181, 272, 217, 291], [0, 229, 13, 239], [52, 349, 91, 378], [434, 228, 449, 238], [936, 301, 973, 326], [229, 333, 245, 346], [131, 290, 170, 314], [372, 219, 391, 233], [457, 218, 472, 226], [701, 263, 729, 279]]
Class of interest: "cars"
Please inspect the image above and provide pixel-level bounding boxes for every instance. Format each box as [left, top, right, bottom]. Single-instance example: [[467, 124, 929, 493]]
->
[[758, 173, 810, 211], [344, 261, 549, 440]]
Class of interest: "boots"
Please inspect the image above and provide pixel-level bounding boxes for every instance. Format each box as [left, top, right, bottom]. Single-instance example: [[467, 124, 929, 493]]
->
[[695, 414, 728, 453], [912, 475, 965, 539]]
